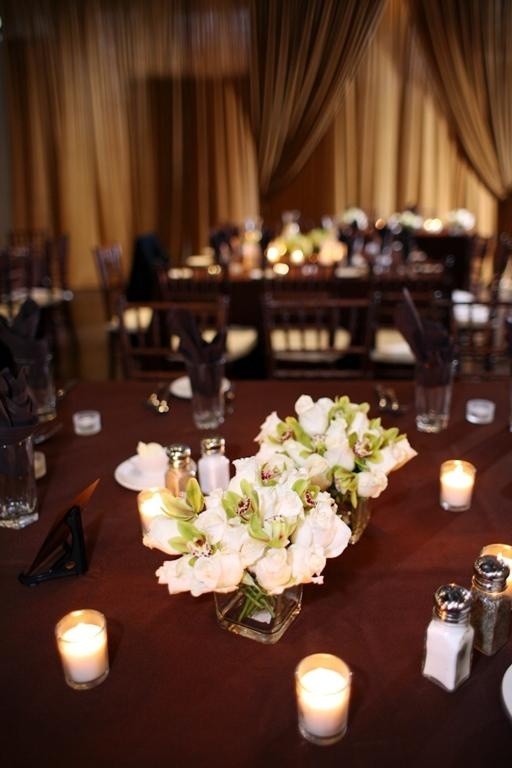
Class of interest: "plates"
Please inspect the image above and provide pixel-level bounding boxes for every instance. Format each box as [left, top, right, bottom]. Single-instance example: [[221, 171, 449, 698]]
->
[[114, 450, 197, 493]]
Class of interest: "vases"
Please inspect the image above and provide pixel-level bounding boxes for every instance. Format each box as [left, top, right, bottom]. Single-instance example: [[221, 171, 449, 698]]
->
[[208, 578, 307, 646]]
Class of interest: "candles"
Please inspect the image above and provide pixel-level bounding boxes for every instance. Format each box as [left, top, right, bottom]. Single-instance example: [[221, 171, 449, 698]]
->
[[288, 651, 357, 749], [475, 539, 511, 608], [51, 599, 115, 696], [433, 451, 478, 519]]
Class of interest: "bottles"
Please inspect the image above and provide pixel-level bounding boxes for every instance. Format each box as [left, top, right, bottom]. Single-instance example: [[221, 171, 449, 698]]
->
[[422, 583, 474, 696], [197, 437, 231, 496], [470, 555, 512, 658], [165, 444, 197, 497]]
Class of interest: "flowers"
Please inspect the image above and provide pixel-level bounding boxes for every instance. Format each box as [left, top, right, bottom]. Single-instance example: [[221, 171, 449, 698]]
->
[[114, 390, 416, 604]]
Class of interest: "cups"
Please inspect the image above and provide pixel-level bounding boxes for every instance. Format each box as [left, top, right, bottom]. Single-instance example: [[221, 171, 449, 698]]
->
[[294, 652, 353, 747], [186, 355, 229, 431], [439, 460, 477, 513], [0, 354, 63, 446], [54, 609, 112, 691], [1, 430, 41, 531], [413, 360, 458, 433]]
